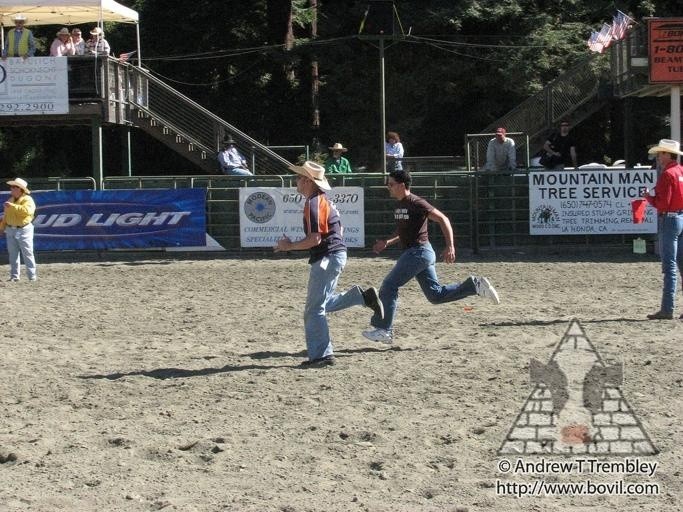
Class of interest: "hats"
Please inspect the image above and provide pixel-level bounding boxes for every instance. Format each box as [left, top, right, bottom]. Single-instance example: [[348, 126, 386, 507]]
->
[[645, 137, 683, 157], [328, 142, 349, 153], [221, 135, 237, 144], [53, 26, 105, 38], [10, 14, 28, 24], [286, 160, 333, 192], [5, 177, 31, 195], [495, 127, 507, 136]]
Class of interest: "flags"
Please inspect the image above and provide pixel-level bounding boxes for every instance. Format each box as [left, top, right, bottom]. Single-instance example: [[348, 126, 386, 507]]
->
[[588, 14, 630, 54]]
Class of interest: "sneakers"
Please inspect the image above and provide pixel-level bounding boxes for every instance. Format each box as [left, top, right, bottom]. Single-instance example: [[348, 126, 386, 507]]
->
[[302, 354, 338, 369], [646, 308, 675, 321], [360, 284, 387, 322], [476, 276, 502, 306], [361, 326, 395, 345]]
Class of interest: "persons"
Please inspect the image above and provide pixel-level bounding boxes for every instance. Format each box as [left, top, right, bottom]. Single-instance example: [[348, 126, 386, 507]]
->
[[0, 177, 36, 281], [577, 127, 615, 167], [361, 169, 501, 341], [1, 12, 110, 58], [274, 160, 385, 367], [543, 122, 579, 169], [642, 140, 683, 320], [386, 131, 405, 170], [484, 127, 517, 171], [217, 135, 253, 175], [322, 142, 353, 181]]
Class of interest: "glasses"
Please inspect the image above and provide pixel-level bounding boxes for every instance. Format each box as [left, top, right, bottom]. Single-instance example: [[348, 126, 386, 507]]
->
[[387, 182, 401, 189]]
